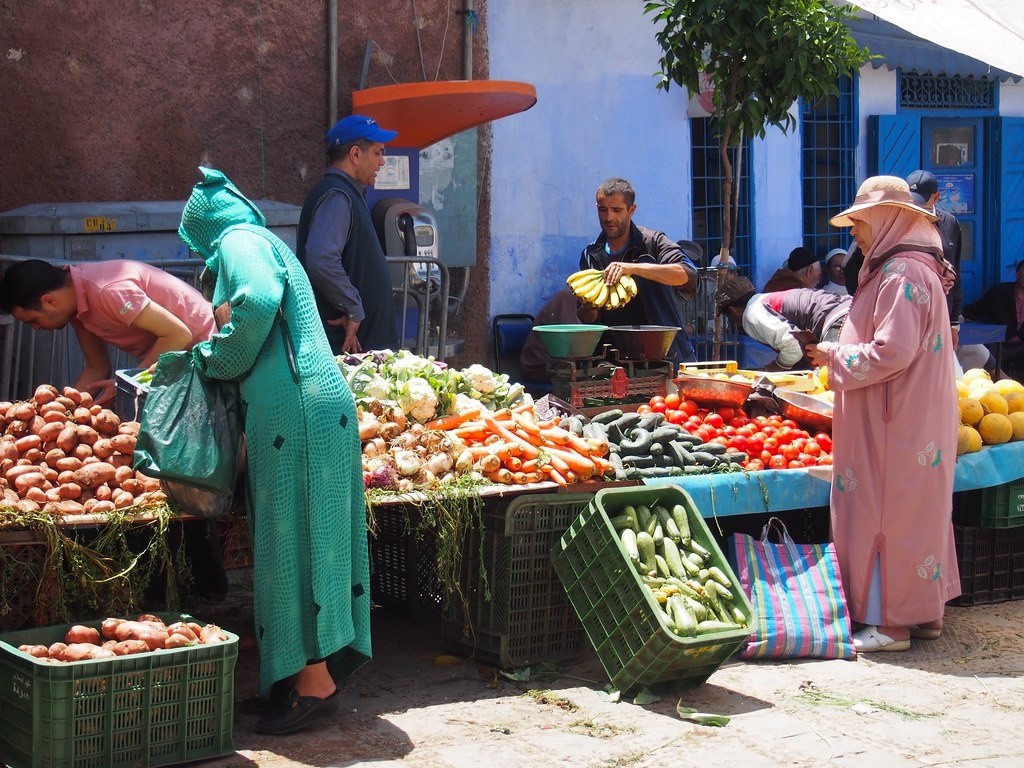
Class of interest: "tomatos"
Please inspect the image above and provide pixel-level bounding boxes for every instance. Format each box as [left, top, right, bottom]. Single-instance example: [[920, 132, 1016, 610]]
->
[[639, 393, 833, 473]]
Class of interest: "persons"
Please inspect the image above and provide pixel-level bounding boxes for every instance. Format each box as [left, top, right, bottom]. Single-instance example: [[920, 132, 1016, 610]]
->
[[716, 275, 852, 372], [146, 165, 372, 734], [0, 258, 229, 601], [674, 239, 727, 338], [296, 114, 397, 353], [957, 260, 1024, 386], [841, 170, 962, 350], [806, 176, 963, 651], [823, 248, 847, 295], [519, 179, 696, 384], [761, 248, 820, 292]]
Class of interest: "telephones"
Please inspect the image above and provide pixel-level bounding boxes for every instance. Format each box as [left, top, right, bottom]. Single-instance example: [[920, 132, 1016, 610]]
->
[[374, 198, 442, 302]]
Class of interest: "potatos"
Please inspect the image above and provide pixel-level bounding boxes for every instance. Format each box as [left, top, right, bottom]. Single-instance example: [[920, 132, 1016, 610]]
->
[[0, 385, 171, 513], [17, 612, 229, 757]]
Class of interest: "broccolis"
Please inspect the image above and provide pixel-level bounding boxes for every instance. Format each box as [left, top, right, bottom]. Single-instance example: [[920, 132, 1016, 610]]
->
[[336, 351, 522, 423]]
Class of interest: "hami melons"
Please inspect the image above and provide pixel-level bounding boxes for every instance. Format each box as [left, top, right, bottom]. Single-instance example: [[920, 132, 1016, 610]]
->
[[948, 367, 1024, 457]]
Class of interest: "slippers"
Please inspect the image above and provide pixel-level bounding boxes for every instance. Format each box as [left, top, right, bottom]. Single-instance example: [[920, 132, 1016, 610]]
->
[[910, 624, 941, 641], [850, 625, 912, 652]]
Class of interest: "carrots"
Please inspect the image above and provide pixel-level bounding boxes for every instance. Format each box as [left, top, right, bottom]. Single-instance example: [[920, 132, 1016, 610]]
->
[[423, 404, 614, 490]]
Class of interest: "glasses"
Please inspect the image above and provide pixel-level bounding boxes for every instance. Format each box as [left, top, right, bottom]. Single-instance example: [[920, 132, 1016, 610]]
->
[[829, 264, 841, 271]]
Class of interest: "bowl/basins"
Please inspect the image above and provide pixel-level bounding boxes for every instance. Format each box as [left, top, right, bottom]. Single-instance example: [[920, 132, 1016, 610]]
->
[[672, 378, 756, 407], [533, 324, 608, 358], [773, 388, 833, 431], [609, 326, 682, 359]]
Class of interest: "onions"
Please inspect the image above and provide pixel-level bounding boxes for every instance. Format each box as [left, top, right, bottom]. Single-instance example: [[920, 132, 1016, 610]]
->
[[352, 402, 475, 491]]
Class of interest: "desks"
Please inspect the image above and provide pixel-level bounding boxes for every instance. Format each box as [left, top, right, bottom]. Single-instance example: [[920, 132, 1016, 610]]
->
[[0, 375, 1024, 547]]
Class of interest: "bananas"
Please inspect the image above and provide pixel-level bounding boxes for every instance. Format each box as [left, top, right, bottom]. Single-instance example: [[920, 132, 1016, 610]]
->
[[567, 268, 637, 310]]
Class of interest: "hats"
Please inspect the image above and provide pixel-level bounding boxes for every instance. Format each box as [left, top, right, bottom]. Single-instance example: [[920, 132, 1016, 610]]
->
[[710, 254, 737, 270], [326, 113, 398, 146], [829, 174, 940, 228], [676, 239, 702, 264], [716, 275, 755, 317], [904, 170, 936, 206], [788, 247, 821, 272], [826, 248, 848, 264]]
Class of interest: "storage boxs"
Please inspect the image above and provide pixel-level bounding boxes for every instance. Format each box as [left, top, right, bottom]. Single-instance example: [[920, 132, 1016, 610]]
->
[[0, 484, 1024, 768], [689, 333, 778, 369]]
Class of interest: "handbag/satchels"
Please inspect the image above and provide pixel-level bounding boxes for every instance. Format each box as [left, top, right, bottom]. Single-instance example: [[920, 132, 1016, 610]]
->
[[727, 516, 857, 661], [133, 352, 247, 518]]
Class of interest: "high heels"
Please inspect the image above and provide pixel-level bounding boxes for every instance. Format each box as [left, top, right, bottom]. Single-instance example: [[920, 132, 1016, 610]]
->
[[251, 675, 344, 733]]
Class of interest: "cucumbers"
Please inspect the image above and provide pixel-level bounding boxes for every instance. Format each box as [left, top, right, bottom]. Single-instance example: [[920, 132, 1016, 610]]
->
[[560, 410, 749, 481]]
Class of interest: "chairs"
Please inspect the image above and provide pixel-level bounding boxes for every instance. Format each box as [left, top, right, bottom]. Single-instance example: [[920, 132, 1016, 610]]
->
[[493, 313, 553, 398]]
